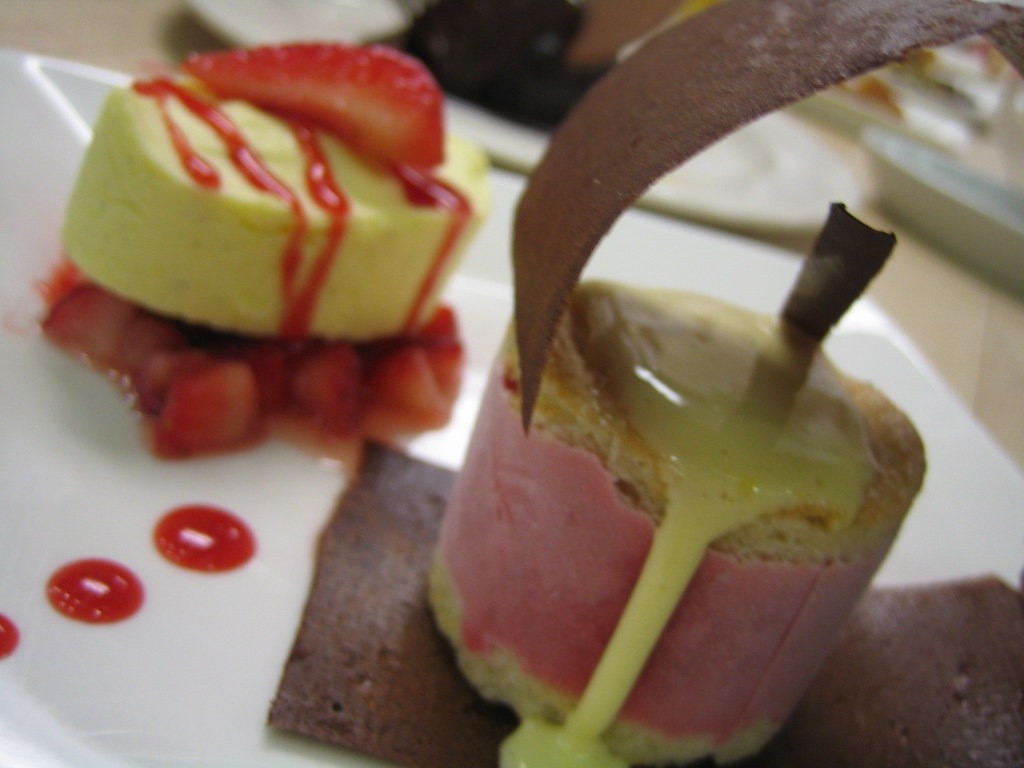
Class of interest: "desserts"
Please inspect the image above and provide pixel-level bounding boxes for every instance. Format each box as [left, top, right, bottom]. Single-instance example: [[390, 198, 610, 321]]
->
[[263, 0, 1024, 768], [35, 42, 494, 460]]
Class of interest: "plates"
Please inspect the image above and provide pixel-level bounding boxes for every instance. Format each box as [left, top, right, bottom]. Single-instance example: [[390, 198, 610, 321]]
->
[[0, 48, 1024, 768], [445, 92, 860, 238]]
